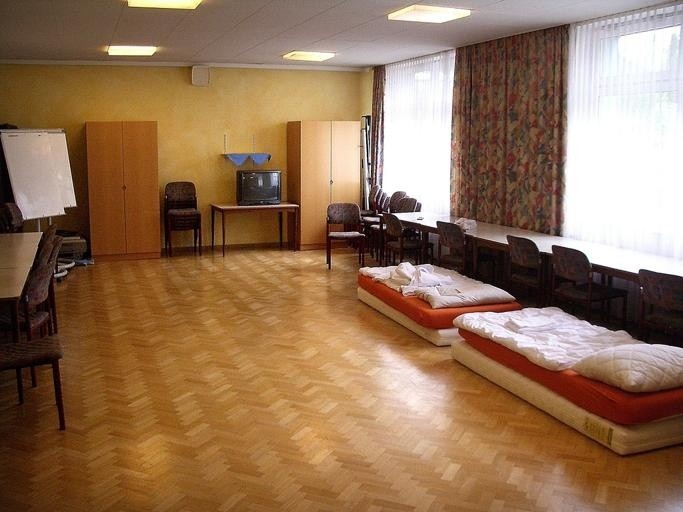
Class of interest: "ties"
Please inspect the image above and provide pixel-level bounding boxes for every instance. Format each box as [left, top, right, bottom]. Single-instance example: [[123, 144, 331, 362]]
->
[[236, 169, 282, 205]]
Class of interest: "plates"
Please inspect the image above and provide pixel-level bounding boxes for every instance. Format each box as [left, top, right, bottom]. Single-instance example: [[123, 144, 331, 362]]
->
[[86, 120, 161, 260], [286, 120, 361, 250]]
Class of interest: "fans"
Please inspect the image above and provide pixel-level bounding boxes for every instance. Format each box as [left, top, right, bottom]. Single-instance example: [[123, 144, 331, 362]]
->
[[0, 127, 78, 221]]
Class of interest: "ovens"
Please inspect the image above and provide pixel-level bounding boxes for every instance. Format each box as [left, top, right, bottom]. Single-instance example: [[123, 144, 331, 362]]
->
[[356, 262, 524, 348]]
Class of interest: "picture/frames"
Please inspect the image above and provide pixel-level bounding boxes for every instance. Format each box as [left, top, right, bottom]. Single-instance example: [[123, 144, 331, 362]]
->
[[638, 266, 682, 344], [552, 244, 629, 327], [210, 201, 300, 257], [378, 211, 683, 324], [437, 219, 496, 280], [506, 233, 546, 306]]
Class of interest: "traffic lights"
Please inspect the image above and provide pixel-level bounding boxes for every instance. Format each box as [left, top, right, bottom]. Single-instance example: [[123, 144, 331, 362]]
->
[[448, 306, 682, 455], [414, 281, 517, 309], [569, 343, 683, 392]]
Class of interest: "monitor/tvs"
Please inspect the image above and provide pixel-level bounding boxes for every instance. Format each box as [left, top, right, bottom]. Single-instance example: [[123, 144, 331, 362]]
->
[[55, 230, 82, 241]]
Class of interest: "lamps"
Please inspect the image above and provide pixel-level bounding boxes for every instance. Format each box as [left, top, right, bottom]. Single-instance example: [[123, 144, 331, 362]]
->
[[165, 181, 203, 257], [0, 223, 68, 432], [350, 185, 433, 266], [382, 213, 433, 264], [326, 203, 365, 270]]
